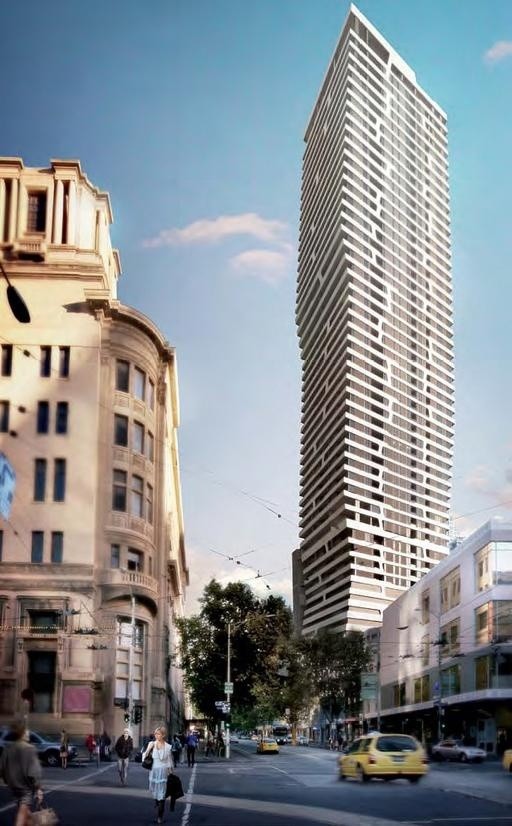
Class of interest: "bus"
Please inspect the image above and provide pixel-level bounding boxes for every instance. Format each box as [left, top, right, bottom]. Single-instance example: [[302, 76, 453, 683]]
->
[[261, 721, 287, 744]]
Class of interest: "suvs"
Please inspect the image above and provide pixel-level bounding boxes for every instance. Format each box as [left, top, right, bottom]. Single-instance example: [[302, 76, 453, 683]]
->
[[0, 729, 79, 768]]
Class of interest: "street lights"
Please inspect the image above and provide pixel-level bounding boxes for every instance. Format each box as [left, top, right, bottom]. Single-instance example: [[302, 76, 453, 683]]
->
[[116, 567, 137, 753], [222, 615, 276, 759], [411, 608, 442, 741]]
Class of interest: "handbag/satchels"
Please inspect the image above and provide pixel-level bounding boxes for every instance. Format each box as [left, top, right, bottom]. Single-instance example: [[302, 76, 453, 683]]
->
[[31, 808, 59, 826], [141, 755, 153, 770]]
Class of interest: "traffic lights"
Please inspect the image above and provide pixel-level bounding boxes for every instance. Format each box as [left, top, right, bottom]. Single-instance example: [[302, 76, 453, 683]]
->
[[124, 714, 130, 723], [134, 705, 143, 725], [225, 714, 232, 730]]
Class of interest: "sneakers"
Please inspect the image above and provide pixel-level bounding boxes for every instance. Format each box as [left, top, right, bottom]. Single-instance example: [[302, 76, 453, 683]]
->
[[121, 782, 128, 785], [158, 818, 163, 824], [174, 763, 193, 768]]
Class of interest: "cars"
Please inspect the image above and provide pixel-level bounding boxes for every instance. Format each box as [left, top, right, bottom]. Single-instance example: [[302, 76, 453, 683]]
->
[[257, 738, 280, 754], [433, 740, 487, 764], [337, 732, 430, 784], [502, 750, 512, 774], [230, 736, 239, 744], [287, 735, 310, 747]]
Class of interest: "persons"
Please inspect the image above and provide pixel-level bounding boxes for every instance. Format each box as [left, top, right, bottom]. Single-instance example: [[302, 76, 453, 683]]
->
[[169, 729, 225, 769], [1, 723, 44, 826], [114, 728, 134, 787], [60, 728, 71, 770], [84, 730, 113, 763], [141, 726, 174, 826]]
